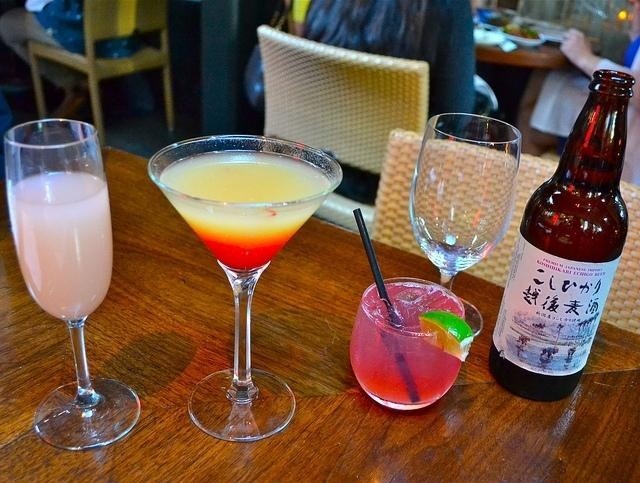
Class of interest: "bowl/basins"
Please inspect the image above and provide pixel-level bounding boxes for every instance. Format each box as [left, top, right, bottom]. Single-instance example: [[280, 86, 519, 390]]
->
[[474, 6, 571, 50]]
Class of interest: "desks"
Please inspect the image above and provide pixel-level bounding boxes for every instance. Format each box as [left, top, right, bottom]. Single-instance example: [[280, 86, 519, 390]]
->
[[1, 146, 639, 482]]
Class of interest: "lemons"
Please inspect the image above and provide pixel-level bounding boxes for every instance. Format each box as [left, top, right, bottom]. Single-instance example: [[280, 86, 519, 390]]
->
[[419, 310, 475, 363]]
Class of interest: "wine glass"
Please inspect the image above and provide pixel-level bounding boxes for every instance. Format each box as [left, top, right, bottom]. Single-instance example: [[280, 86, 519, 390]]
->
[[146, 133, 343, 441], [407, 111, 521, 349], [3, 118, 142, 449]]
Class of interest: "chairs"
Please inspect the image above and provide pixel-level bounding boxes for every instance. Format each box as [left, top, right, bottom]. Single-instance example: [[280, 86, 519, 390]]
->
[[255, 25, 431, 237], [369, 123, 640, 336], [26, 0, 174, 145]]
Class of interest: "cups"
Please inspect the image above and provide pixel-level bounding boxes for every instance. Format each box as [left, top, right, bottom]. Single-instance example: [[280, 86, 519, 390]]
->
[[350, 276, 467, 415]]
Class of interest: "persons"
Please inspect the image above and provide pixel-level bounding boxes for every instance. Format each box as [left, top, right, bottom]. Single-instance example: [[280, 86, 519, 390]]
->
[[291, 0, 315, 40], [301, 0, 499, 132], [0, 0, 142, 119], [512, 0, 640, 183]]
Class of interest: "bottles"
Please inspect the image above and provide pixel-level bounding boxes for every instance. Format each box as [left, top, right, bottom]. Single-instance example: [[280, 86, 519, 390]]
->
[[486, 66, 636, 402]]
